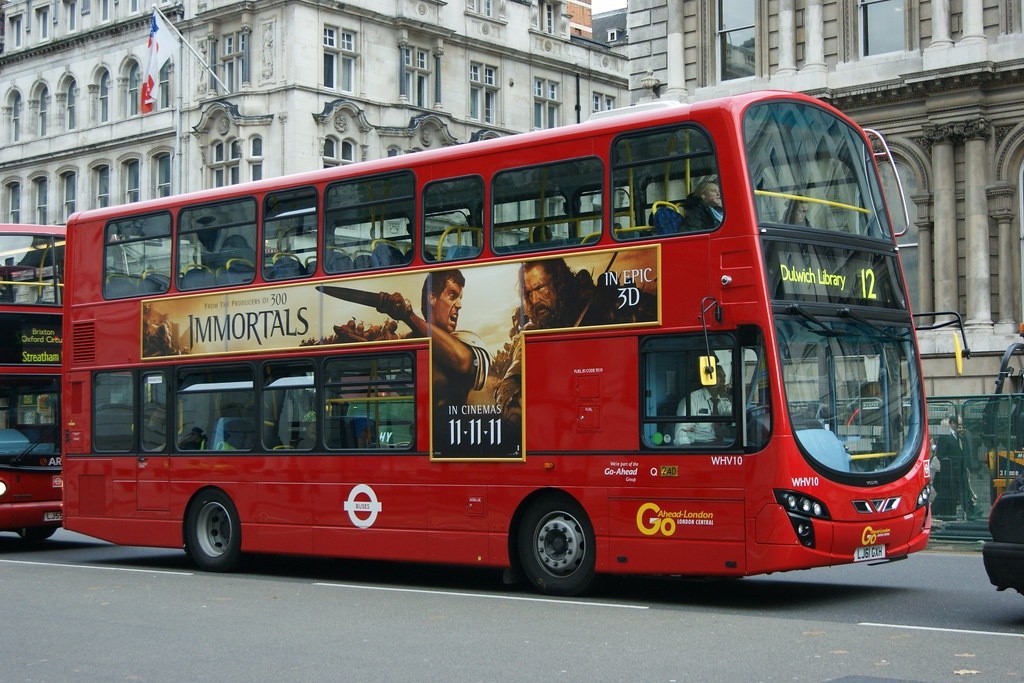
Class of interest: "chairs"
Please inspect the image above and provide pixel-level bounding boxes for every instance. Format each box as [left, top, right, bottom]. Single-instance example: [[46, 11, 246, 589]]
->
[[210, 414, 381, 453], [107, 198, 690, 297]]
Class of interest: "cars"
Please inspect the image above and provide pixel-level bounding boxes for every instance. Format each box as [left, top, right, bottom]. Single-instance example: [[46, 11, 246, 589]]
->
[[982, 491, 1024, 596]]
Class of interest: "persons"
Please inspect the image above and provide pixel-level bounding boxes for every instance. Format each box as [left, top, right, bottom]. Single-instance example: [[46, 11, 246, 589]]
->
[[929, 415, 983, 521], [679, 180, 724, 232], [376, 268, 492, 455], [494, 259, 609, 443], [673, 364, 734, 447], [776, 194, 812, 227]]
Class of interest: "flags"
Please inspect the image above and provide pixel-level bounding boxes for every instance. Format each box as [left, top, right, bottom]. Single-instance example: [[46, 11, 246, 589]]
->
[[139, 8, 178, 112]]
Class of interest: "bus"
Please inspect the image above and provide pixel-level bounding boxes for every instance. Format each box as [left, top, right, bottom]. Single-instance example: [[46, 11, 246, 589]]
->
[[59, 89, 974, 596], [0, 222, 67, 542]]
